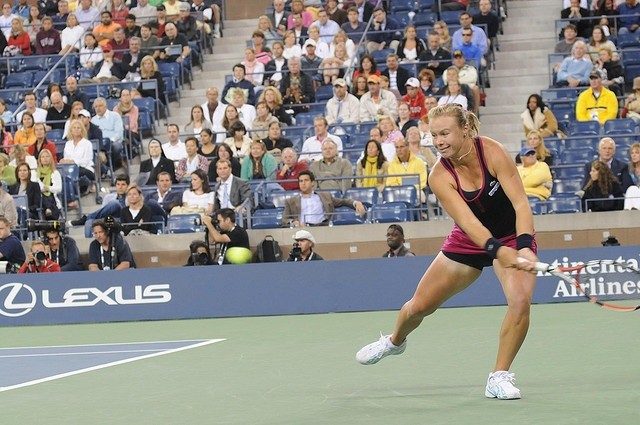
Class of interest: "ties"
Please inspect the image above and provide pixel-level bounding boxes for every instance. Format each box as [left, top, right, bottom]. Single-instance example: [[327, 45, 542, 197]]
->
[[222, 184, 229, 209]]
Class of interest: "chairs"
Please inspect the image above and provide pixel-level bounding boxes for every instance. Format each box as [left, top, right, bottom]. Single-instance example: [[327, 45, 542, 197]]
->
[[347, 31, 365, 44], [552, 100, 573, 120], [47, 120, 65, 128], [606, 35, 617, 45], [164, 62, 180, 93], [164, 45, 182, 55], [540, 89, 577, 101], [622, 47, 640, 64], [392, 10, 437, 26], [1, 88, 36, 103], [351, 134, 375, 150], [57, 163, 83, 217], [468, 60, 483, 94], [569, 120, 600, 134], [139, 184, 189, 197], [50, 69, 76, 81], [4, 74, 34, 88], [360, 119, 379, 133], [98, 83, 112, 95], [45, 129, 66, 137], [388, 0, 435, 11], [8, 56, 26, 70], [6, 103, 21, 112], [54, 142, 65, 152], [114, 83, 141, 91], [296, 112, 320, 125], [314, 84, 335, 101], [143, 79, 165, 124], [35, 69, 50, 84], [285, 135, 301, 149], [283, 126, 313, 141], [160, 63, 174, 114], [134, 98, 157, 134], [345, 185, 378, 204], [78, 67, 94, 79], [120, 223, 164, 234], [551, 196, 581, 212], [41, 87, 68, 96], [625, 65, 640, 81], [48, 56, 67, 66], [140, 46, 159, 56], [549, 53, 569, 86], [37, 167, 67, 221], [304, 34, 334, 45], [252, 207, 285, 226], [522, 136, 559, 153], [467, 8, 497, 52], [416, 60, 451, 73], [382, 186, 417, 202], [25, 56, 50, 69], [165, 214, 202, 232], [121, 116, 133, 172], [375, 61, 414, 75], [606, 130, 633, 134], [189, 180, 216, 192], [370, 202, 413, 221], [553, 177, 585, 195], [448, 24, 490, 80], [560, 148, 597, 163], [1, 58, 9, 67], [329, 123, 357, 138], [400, 27, 428, 41], [89, 139, 101, 190], [551, 164, 586, 176], [366, 32, 402, 41], [564, 135, 599, 148], [66, 55, 78, 74], [105, 100, 119, 110], [618, 33, 640, 45], [138, 114, 144, 156], [556, 121, 568, 133], [181, 49, 195, 86], [329, 205, 362, 224], [442, 11, 467, 24], [249, 179, 264, 191], [9, 195, 29, 240], [606, 118, 636, 128], [103, 138, 116, 185], [602, 135, 639, 163], [114, 49, 130, 59], [318, 188, 342, 200], [270, 190, 300, 207], [303, 102, 327, 119], [77, 84, 97, 95]]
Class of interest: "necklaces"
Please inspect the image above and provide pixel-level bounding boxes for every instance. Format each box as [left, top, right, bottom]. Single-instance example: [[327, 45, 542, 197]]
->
[[446, 144, 473, 163]]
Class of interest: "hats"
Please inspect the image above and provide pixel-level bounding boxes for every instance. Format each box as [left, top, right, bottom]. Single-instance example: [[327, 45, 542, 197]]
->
[[404, 77, 420, 88], [293, 230, 316, 248], [390, 224, 405, 243], [519, 147, 537, 156], [453, 50, 463, 58], [305, 38, 317, 48], [590, 70, 601, 78], [180, 2, 190, 10], [102, 44, 112, 51], [633, 76, 640, 89], [367, 75, 380, 85], [156, 4, 165, 10], [333, 78, 347, 88], [78, 108, 91, 118]]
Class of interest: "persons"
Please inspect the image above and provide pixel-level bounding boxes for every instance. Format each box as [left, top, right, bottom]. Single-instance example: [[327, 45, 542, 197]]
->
[[521, 94, 558, 139], [515, 131, 554, 177], [353, 75, 368, 99], [281, 169, 365, 228], [258, 16, 285, 48], [258, 85, 292, 125], [220, 88, 255, 137], [207, 0, 221, 38], [621, 142, 640, 193], [434, 20, 451, 51], [419, 115, 433, 146], [28, 123, 58, 164], [174, 138, 209, 182], [353, 55, 382, 84], [241, 141, 277, 181], [0, 116, 13, 154], [562, 0, 588, 9], [309, 139, 354, 191], [582, 137, 633, 195], [0, 98, 15, 136], [66, 33, 105, 84], [158, 22, 191, 81], [221, 105, 247, 138], [46, 91, 71, 130], [251, 31, 274, 66], [200, 86, 228, 142], [366, 7, 399, 57], [45, 227, 86, 272], [30, 149, 63, 220], [381, 224, 415, 258], [329, 30, 355, 56], [471, 0, 500, 51], [139, 138, 179, 185], [259, 122, 293, 163], [581, 162, 625, 213], [59, 119, 96, 208], [621, 77, 640, 125], [262, 41, 289, 85], [270, 0, 292, 32], [324, 0, 350, 27], [189, 0, 213, 35], [396, 102, 419, 137], [317, 44, 355, 85], [1, 152, 17, 187], [144, 171, 183, 223], [418, 31, 452, 77], [359, 75, 399, 124], [451, 27, 484, 74], [555, 41, 593, 96], [185, 241, 220, 265], [62, 101, 84, 140], [27, 0, 47, 16], [0, 217, 26, 273], [381, 75, 402, 103], [302, 25, 329, 57], [240, 49, 265, 94], [185, 106, 212, 144], [418, 68, 438, 97], [430, 83, 440, 99], [623, 162, 640, 211], [420, 94, 440, 122], [443, 51, 480, 115], [356, 103, 539, 399], [121, 36, 150, 82], [0, 2, 21, 38], [288, 231, 323, 262], [615, 0, 640, 51], [225, 122, 254, 158], [438, 78, 467, 110], [278, 56, 315, 112], [575, 71, 618, 126], [139, 25, 160, 60], [382, 54, 412, 97], [300, 40, 323, 87], [325, 78, 360, 125], [555, 24, 587, 57], [379, 115, 406, 149], [201, 209, 250, 266], [16, 92, 48, 131], [593, 48, 627, 108], [386, 138, 427, 204], [87, 219, 136, 272], [90, 97, 124, 179], [75, 0, 101, 34], [197, 128, 219, 161], [90, 44, 123, 83], [559, 0, 590, 41], [42, 83, 68, 111], [438, 66, 474, 110], [70, 173, 130, 226], [124, 14, 141, 38], [248, 102, 278, 142], [147, 4, 175, 41], [275, 147, 311, 192], [111, 0, 129, 28], [305, 7, 322, 23], [8, 163, 41, 228], [590, 0, 620, 35], [92, 11, 121, 48], [65, 76, 89, 115], [120, 186, 151, 236], [17, 242, 60, 274], [123, 0, 136, 9], [288, 0, 312, 31], [23, 5, 43, 44], [299, 116, 344, 164], [515, 145, 553, 216], [35, 18, 62, 55], [13, 112, 38, 150], [129, 1, 158, 27], [586, 26, 621, 68], [107, 27, 129, 63], [354, 139, 388, 191], [180, 168, 215, 222], [396, 26, 425, 64], [77, 109, 103, 155], [208, 142, 240, 181], [210, 159, 253, 230], [162, 0, 183, 19], [1, 180, 19, 234], [9, 144, 38, 170], [289, 13, 308, 45], [311, 10, 341, 48], [344, 0, 375, 22], [113, 88, 140, 154], [282, 31, 302, 59], [341, 7, 370, 34], [130, 55, 167, 105], [50, 1, 71, 30], [221, 64, 256, 106], [60, 14, 84, 54], [161, 124, 188, 168], [6, 18, 30, 56], [399, 77, 428, 120], [12, 0, 31, 18], [173, 2, 200, 60], [451, 13, 489, 73], [358, 126, 396, 164], [406, 126, 436, 220], [1, 29, 8, 55]]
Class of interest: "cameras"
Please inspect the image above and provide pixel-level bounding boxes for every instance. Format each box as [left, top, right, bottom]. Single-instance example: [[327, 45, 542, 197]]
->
[[193, 212, 218, 226], [104, 215, 120, 234], [191, 252, 210, 264], [31, 251, 49, 264], [289, 243, 302, 261]]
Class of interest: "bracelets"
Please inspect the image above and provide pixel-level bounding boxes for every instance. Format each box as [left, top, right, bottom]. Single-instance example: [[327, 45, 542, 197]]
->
[[484, 237, 503, 260], [516, 233, 534, 250]]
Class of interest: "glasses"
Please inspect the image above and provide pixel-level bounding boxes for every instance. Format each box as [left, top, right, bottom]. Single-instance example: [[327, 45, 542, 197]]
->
[[462, 33, 471, 36], [526, 151, 535, 156]]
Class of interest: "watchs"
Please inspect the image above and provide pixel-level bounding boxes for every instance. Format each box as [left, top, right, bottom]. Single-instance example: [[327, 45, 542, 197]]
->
[[180, 54, 186, 59]]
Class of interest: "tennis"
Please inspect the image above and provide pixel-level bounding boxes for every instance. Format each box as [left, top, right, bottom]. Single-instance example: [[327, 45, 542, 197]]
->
[[226, 247, 251, 265]]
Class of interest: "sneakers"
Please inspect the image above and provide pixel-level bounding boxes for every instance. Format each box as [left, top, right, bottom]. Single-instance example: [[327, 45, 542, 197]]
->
[[355, 330, 408, 366], [484, 371, 522, 400]]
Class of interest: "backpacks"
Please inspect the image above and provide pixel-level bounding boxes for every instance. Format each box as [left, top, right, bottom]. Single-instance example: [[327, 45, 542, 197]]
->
[[252, 235, 282, 262]]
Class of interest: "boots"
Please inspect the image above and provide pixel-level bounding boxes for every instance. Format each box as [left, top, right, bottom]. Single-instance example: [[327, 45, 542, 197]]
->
[[68, 215, 88, 226]]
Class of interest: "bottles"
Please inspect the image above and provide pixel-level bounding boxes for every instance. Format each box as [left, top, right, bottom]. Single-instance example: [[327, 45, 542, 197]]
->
[[276, 209, 283, 226]]
